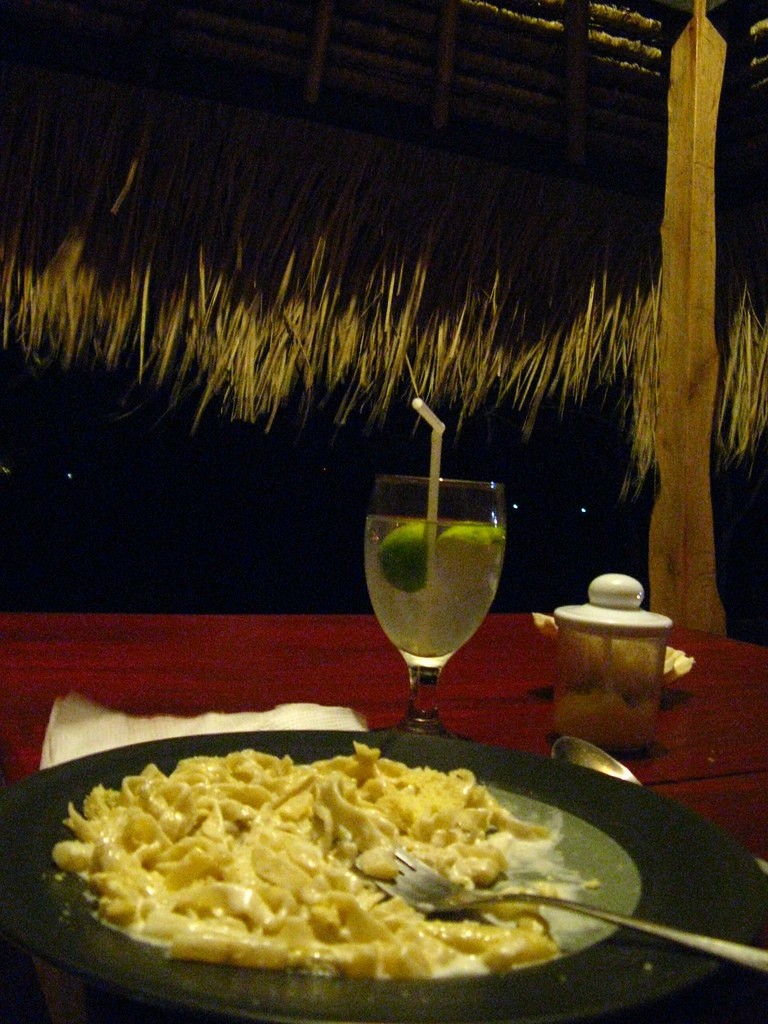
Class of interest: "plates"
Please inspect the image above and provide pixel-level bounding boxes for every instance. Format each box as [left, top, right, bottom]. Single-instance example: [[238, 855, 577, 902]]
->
[[0, 727, 767, 1024]]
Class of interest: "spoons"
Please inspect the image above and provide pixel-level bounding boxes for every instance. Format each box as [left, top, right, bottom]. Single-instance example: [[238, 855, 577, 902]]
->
[[551, 733, 644, 793]]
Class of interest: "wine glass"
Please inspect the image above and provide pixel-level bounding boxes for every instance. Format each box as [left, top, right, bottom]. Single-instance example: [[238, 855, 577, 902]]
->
[[362, 472, 509, 738]]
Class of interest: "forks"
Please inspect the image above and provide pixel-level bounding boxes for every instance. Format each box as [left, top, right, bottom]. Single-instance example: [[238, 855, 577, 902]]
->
[[354, 842, 768, 980]]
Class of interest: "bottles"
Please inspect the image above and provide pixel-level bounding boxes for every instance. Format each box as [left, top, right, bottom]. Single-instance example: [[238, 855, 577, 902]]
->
[[552, 573, 674, 752]]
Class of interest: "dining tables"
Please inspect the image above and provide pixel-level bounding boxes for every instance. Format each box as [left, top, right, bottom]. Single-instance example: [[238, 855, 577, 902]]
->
[[0, 613, 768, 1023]]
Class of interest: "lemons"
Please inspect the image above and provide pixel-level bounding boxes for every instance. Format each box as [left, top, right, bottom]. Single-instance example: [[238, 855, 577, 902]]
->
[[378, 523, 428, 594], [436, 524, 505, 596]]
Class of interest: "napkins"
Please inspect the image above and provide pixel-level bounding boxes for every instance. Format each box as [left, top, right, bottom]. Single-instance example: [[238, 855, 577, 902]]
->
[[39, 692, 367, 771]]
